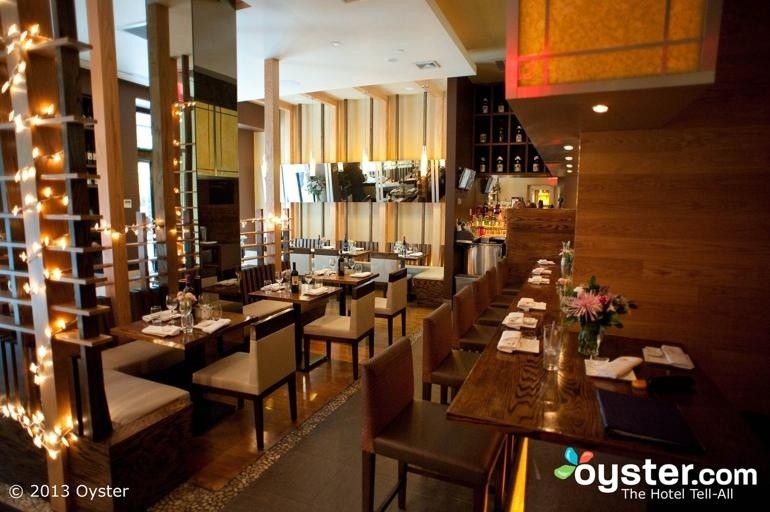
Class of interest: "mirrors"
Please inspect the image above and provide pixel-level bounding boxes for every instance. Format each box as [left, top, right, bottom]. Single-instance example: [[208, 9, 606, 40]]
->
[[279, 156, 447, 204], [198, 178, 242, 280], [168, 0, 195, 103], [193, 1, 238, 111]]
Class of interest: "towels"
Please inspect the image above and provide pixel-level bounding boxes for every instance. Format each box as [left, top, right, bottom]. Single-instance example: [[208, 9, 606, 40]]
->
[[497, 257, 550, 356], [141, 309, 177, 322], [140, 324, 180, 336], [203, 317, 231, 336], [591, 341, 692, 380]]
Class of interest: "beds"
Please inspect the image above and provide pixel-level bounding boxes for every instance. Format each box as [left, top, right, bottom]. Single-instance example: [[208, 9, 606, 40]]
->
[[110, 306, 258, 440]]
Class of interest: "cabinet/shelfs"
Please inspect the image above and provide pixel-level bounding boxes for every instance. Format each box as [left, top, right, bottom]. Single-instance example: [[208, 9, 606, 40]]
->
[[473, 83, 554, 178]]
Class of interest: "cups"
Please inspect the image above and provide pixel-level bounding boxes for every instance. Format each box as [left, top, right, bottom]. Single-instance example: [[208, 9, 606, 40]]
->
[[150, 304, 223, 334], [264, 279, 273, 297], [413, 244, 419, 254], [542, 323, 562, 372]]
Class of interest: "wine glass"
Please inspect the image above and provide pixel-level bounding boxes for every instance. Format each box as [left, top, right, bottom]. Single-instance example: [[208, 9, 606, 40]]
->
[[198, 291, 211, 327], [164, 293, 180, 330], [305, 256, 363, 296], [275, 269, 285, 294]]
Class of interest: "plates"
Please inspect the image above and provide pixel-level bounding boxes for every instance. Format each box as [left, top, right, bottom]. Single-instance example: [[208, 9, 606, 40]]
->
[[642, 347, 694, 370], [393, 249, 404, 254], [410, 252, 424, 257], [499, 260, 557, 354], [584, 358, 639, 383], [352, 246, 365, 251]]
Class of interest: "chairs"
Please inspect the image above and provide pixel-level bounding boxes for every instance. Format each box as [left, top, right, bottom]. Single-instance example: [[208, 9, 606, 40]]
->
[[192, 237, 522, 512]]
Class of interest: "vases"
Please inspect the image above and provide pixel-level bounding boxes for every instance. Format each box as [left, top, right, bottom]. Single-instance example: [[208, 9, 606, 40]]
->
[[560, 256, 570, 278], [180, 309, 194, 334], [575, 320, 605, 357]]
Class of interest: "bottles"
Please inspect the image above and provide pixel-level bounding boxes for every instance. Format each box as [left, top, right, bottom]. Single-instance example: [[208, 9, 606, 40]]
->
[[477, 90, 541, 172], [343, 233, 350, 251], [465, 204, 506, 238], [290, 261, 300, 294], [337, 249, 346, 277], [182, 273, 195, 299], [402, 235, 407, 257], [317, 234, 322, 248]]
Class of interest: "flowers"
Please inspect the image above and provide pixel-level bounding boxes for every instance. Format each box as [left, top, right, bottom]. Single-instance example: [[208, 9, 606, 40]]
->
[[557, 239, 576, 257], [561, 273, 638, 329], [176, 290, 196, 311], [305, 173, 326, 199]]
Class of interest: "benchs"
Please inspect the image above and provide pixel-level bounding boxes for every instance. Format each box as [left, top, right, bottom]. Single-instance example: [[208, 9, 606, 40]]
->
[[1, 338, 186, 511]]
